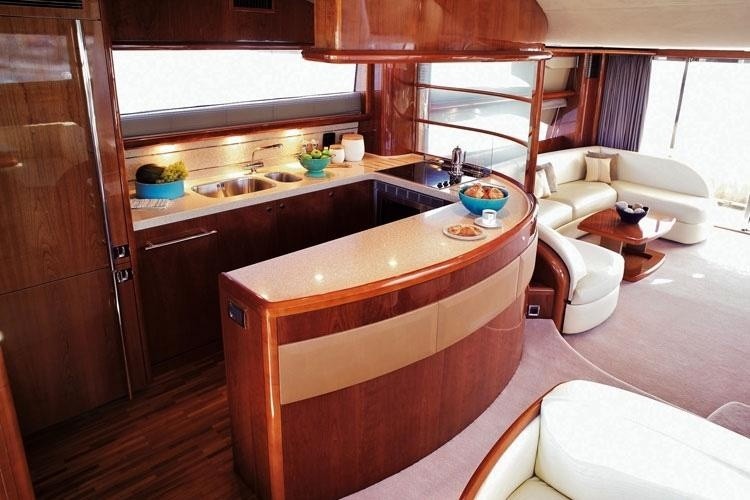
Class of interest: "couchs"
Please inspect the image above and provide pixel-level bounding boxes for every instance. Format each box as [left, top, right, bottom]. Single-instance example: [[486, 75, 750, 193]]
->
[[485, 145, 714, 333]]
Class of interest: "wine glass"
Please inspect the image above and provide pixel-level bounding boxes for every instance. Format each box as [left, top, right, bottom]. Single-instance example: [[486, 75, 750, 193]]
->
[[299, 158, 331, 178]]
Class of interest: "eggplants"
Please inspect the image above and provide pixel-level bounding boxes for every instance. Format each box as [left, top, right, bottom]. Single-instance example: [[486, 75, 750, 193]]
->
[[135, 164, 163, 184]]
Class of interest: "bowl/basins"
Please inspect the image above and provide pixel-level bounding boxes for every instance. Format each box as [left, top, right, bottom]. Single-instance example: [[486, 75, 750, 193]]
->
[[615, 205, 649, 223], [459, 184, 509, 215]]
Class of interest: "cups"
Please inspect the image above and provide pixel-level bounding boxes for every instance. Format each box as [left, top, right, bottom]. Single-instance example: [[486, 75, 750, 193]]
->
[[482, 209, 497, 225]]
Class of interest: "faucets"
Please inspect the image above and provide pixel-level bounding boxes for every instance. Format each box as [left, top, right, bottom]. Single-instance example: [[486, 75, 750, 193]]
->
[[251, 143, 282, 171]]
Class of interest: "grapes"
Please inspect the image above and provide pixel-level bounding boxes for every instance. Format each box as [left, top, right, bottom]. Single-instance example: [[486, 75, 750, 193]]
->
[[155, 159, 189, 184]]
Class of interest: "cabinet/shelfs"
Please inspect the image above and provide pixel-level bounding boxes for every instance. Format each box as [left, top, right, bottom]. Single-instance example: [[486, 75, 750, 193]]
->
[[134, 179, 438, 388]]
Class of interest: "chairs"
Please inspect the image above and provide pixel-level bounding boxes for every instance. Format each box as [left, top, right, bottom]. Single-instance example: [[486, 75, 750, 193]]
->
[[457, 378, 750, 500]]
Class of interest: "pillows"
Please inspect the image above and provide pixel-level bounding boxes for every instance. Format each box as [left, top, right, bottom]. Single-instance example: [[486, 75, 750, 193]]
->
[[584, 150, 620, 185], [534, 162, 558, 198]]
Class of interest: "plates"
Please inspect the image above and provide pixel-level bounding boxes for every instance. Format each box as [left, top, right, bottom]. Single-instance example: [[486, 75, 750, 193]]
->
[[443, 225, 487, 240], [475, 217, 502, 228]]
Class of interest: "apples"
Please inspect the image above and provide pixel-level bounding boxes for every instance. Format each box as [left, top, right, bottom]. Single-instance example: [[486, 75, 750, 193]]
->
[[301, 149, 331, 160]]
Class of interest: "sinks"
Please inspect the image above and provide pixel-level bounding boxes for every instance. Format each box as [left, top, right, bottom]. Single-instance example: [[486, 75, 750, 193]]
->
[[191, 176, 276, 199], [266, 171, 303, 183]]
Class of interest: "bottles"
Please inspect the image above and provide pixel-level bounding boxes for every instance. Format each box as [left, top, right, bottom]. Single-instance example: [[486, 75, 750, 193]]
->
[[322, 146, 329, 154], [329, 134, 364, 164]]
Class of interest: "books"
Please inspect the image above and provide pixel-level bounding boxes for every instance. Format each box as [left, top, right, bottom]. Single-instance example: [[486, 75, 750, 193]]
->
[[128, 198, 169, 209]]
